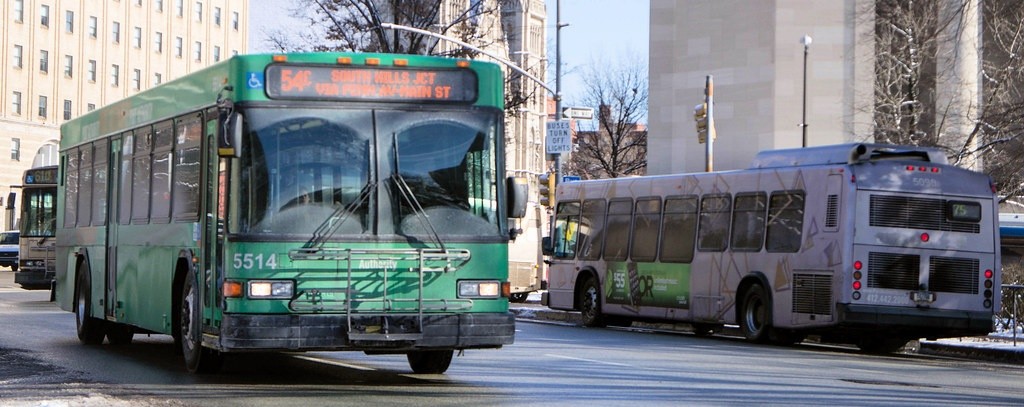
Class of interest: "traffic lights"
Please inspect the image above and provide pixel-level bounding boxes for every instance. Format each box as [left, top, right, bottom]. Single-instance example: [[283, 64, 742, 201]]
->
[[694, 99, 706, 144], [538, 171, 554, 208]]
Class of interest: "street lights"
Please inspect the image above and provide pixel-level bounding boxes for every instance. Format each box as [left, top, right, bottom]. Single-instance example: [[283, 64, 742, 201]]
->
[[382, 0, 567, 306]]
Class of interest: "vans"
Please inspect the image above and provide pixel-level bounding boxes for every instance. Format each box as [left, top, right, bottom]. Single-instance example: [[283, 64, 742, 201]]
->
[[0, 229, 51, 271]]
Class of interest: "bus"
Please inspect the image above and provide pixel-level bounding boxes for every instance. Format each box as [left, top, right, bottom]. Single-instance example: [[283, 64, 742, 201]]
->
[[6, 165, 61, 299], [542, 143, 1003, 346], [51, 50, 528, 373]]
